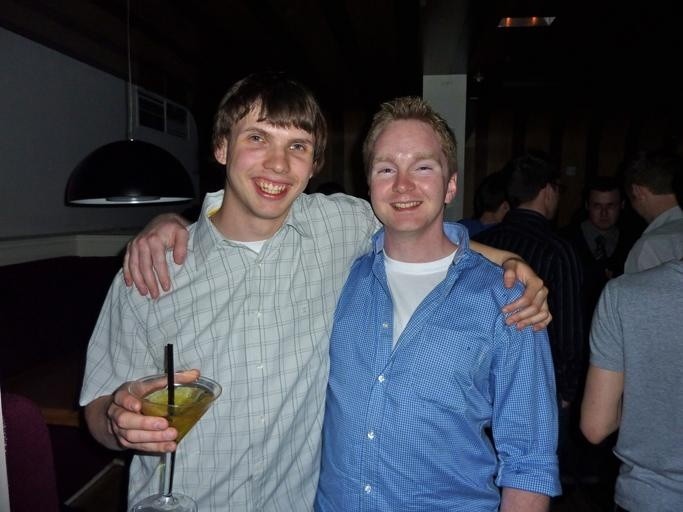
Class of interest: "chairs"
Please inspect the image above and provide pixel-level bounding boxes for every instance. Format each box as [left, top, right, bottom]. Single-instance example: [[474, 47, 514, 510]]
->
[[0, 388, 125, 512]]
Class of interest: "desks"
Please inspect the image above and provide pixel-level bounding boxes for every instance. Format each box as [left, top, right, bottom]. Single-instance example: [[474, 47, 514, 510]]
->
[[2, 357, 86, 426]]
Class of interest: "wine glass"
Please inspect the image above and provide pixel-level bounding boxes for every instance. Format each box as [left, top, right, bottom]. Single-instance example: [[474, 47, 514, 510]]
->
[[126, 372, 222, 511]]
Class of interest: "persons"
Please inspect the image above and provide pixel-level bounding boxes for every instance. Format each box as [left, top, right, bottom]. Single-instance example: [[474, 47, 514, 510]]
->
[[574, 170, 641, 300], [121, 96, 563, 512], [580, 161, 682, 511], [78, 72, 554, 512], [470, 144, 595, 408], [457, 171, 512, 237], [624, 149, 683, 275]]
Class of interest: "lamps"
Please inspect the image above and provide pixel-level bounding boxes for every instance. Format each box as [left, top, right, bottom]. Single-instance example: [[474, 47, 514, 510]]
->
[[63, 0, 195, 207]]
